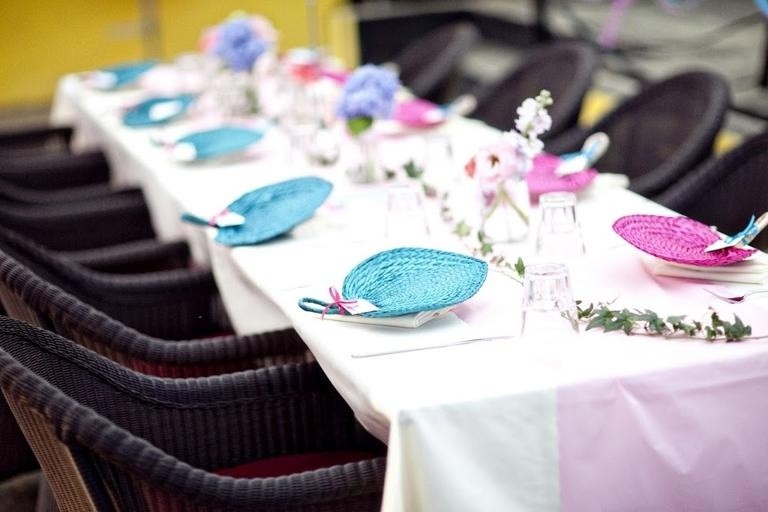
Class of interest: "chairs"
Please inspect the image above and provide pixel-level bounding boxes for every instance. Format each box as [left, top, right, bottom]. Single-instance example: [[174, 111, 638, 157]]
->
[[464, 44, 597, 144], [392, 24, 472, 101], [550, 68, 731, 204], [0, 318, 387, 510], [0, 121, 315, 377], [656, 131, 765, 252]]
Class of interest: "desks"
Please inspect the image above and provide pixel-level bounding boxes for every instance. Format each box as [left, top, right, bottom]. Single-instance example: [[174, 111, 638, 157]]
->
[[61, 55, 766, 510]]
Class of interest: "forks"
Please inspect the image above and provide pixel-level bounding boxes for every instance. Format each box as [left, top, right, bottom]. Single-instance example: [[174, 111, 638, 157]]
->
[[704, 285, 768, 305]]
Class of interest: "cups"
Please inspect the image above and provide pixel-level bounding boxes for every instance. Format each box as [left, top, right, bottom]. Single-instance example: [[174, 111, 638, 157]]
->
[[520, 265, 580, 343], [536, 191, 587, 263]]
